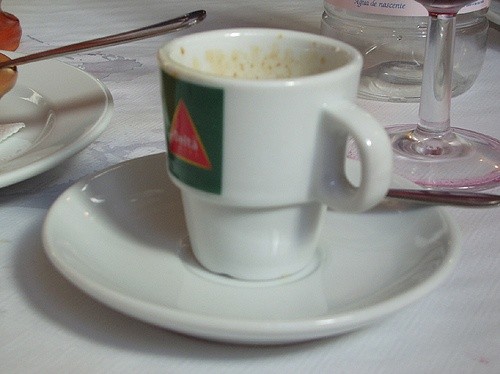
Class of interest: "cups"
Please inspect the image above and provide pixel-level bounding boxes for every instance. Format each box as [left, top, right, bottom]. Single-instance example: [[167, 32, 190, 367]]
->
[[156, 28, 395, 281]]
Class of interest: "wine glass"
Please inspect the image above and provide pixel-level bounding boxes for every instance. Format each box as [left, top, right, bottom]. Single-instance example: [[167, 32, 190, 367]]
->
[[347, 0, 500, 193]]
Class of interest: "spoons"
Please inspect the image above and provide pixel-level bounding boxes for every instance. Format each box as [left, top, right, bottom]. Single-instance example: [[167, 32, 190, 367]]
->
[[0, 9, 207, 99]]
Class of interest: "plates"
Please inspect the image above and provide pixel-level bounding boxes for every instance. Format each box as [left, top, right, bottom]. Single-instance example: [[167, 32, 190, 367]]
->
[[41, 151, 463, 345], [0, 48, 115, 188]]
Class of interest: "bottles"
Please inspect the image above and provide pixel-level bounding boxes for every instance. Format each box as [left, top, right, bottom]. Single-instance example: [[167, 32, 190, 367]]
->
[[319, 0, 492, 104]]
